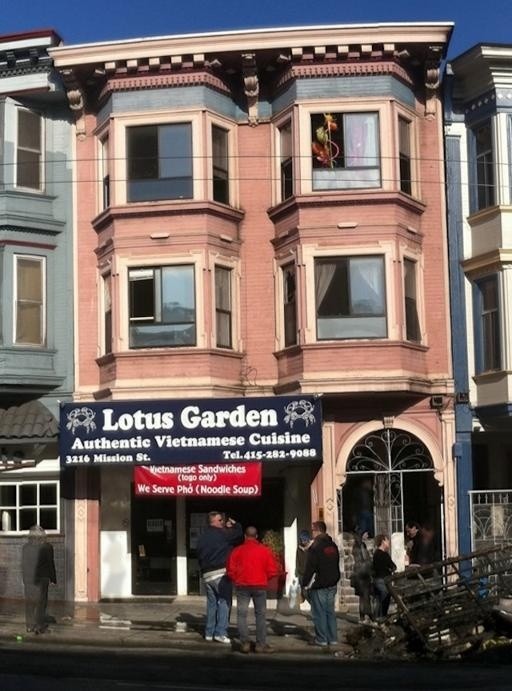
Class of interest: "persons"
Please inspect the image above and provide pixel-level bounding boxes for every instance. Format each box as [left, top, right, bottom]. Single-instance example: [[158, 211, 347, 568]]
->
[[195, 511, 442, 654], [22, 526, 57, 635]]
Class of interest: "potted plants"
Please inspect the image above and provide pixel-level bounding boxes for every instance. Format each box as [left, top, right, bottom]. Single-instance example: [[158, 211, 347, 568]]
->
[[260, 527, 289, 600]]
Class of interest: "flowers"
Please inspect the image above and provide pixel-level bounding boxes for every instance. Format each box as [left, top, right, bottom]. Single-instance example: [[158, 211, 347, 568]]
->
[[312, 110, 341, 171]]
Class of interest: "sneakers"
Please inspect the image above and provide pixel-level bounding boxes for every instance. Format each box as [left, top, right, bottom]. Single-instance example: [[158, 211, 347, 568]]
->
[[308, 640, 317, 645], [204, 635, 212, 641], [240, 642, 250, 653], [213, 636, 230, 644], [255, 645, 274, 654]]
